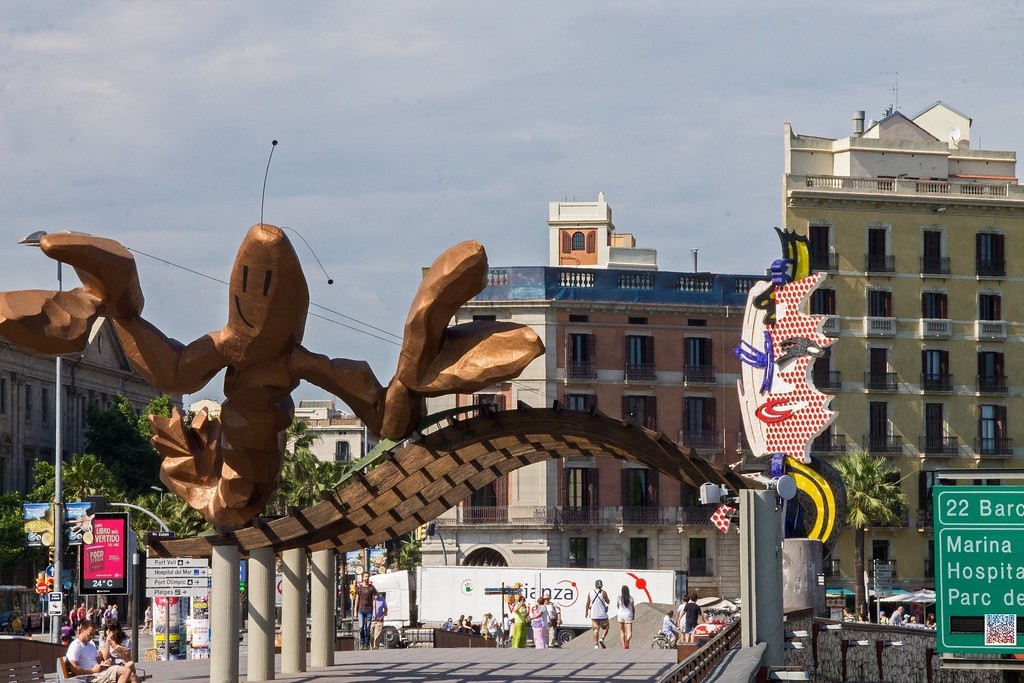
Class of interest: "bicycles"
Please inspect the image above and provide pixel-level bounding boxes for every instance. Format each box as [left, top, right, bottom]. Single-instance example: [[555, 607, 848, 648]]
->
[[651, 632, 676, 649]]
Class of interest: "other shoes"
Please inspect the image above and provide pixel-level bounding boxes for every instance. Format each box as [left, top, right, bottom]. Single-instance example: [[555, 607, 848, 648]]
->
[[594, 645, 599, 650], [623, 640, 630, 649], [359, 644, 364, 650], [599, 638, 607, 649], [364, 644, 368, 650]]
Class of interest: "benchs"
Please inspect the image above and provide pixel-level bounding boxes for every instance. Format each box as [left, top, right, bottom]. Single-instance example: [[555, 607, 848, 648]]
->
[[56, 656, 97, 683], [650, 632, 670, 650]]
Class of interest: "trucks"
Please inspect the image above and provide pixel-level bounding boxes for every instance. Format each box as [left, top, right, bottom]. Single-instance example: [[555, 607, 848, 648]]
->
[[367, 564, 689, 647]]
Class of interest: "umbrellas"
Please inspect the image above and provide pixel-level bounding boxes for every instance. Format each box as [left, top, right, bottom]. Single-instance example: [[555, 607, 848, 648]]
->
[[874, 588, 936, 624]]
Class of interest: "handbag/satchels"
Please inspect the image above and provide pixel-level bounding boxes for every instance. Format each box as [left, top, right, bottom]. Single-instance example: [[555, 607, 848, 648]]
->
[[551, 614, 563, 628]]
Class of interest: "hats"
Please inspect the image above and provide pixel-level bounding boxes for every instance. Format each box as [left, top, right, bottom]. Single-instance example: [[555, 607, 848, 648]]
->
[[904, 614, 911, 619], [595, 580, 603, 588], [517, 595, 526, 601], [879, 610, 885, 616], [543, 594, 550, 599]]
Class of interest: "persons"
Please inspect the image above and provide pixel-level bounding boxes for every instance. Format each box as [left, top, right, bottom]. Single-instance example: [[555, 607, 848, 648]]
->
[[662, 592, 702, 649], [335, 571, 388, 650], [481, 613, 510, 647], [617, 585, 635, 649], [141, 606, 152, 635], [879, 606, 936, 631], [442, 615, 475, 635], [843, 608, 869, 623], [585, 580, 610, 649], [510, 594, 562, 649], [65, 617, 139, 683], [68, 603, 118, 632]]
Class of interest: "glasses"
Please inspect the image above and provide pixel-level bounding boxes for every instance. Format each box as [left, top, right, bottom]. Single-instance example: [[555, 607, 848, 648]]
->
[[81, 630, 96, 636]]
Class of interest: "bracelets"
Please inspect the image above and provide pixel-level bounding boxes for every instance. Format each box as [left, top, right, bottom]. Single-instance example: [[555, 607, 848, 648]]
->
[[471, 628, 472, 630]]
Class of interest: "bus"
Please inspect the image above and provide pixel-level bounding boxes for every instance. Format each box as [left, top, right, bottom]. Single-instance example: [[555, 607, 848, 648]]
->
[[0, 584, 49, 633]]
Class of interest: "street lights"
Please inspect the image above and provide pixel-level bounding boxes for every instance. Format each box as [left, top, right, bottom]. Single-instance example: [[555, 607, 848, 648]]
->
[[15, 230, 92, 644], [151, 485, 162, 533]]
